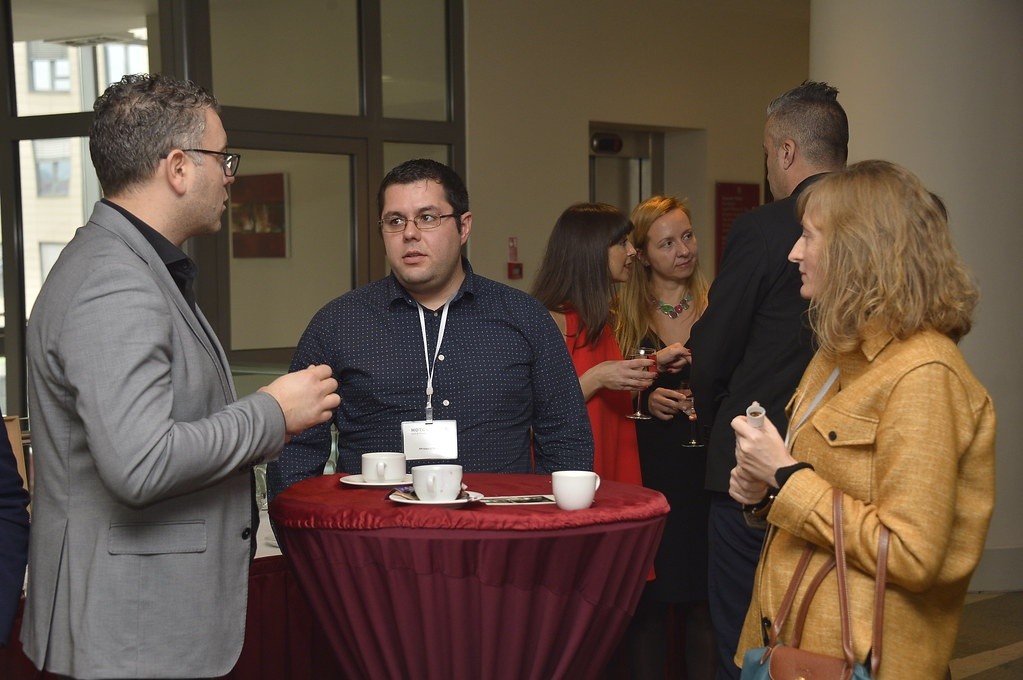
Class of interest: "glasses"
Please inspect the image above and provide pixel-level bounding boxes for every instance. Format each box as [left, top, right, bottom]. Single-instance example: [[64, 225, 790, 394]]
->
[[378, 209, 469, 233], [160, 149, 242, 178]]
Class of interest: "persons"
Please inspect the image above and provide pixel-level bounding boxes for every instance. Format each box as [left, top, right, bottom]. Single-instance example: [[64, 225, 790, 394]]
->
[[529, 202, 692, 582], [690, 81, 947, 680], [264, 158, 595, 504], [605, 194, 712, 680], [19, 72, 341, 680], [729, 159, 996, 680], [0, 412, 31, 648]]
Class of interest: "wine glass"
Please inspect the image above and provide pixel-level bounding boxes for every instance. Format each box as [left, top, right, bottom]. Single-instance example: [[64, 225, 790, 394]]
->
[[679, 383, 707, 447], [626, 347, 658, 420]]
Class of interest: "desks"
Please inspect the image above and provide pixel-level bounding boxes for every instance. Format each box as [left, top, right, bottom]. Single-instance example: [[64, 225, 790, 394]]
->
[[272, 474, 670, 680]]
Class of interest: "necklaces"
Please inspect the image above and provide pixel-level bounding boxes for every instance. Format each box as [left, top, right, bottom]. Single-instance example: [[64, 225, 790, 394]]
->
[[651, 293, 693, 319]]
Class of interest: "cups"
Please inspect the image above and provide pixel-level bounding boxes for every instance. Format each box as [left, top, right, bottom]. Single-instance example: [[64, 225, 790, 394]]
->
[[411, 465, 463, 501], [553, 471, 600, 510], [361, 452, 406, 484]]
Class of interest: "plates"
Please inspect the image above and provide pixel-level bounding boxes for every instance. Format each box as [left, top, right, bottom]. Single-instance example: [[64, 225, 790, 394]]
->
[[389, 491, 484, 509], [339, 474, 413, 488]]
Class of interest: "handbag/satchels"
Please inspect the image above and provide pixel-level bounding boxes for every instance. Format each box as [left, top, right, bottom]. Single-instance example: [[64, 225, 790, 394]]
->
[[741, 487, 892, 680]]
